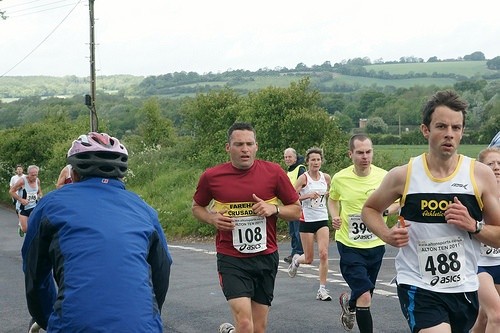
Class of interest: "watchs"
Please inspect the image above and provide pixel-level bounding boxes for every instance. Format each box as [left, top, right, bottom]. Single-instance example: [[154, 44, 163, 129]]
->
[[273, 203, 279, 215], [467, 220, 483, 239], [383, 209, 389, 217]]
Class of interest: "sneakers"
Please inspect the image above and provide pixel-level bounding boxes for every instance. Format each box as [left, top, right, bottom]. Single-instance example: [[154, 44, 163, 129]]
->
[[18, 223, 25, 237], [288, 254, 300, 278], [316, 288, 332, 301], [339, 293, 356, 331]]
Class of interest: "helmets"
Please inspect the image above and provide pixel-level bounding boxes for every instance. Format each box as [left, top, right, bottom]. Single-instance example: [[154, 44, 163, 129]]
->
[[67, 132, 129, 178]]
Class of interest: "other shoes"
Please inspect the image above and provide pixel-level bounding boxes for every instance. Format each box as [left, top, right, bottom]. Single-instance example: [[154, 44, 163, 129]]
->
[[219, 323, 235, 333], [284, 256, 292, 262]]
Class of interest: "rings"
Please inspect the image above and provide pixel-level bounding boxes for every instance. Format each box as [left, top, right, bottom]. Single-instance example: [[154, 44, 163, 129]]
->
[[258, 212, 262, 214]]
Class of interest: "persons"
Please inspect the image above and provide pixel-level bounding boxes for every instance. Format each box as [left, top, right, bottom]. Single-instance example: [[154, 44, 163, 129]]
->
[[361, 90, 500, 333], [328, 134, 401, 333], [192, 122, 301, 333], [283, 148, 307, 264], [469, 148, 500, 333], [56, 163, 73, 189], [9, 165, 43, 237], [21, 132, 172, 333], [288, 146, 333, 301]]
